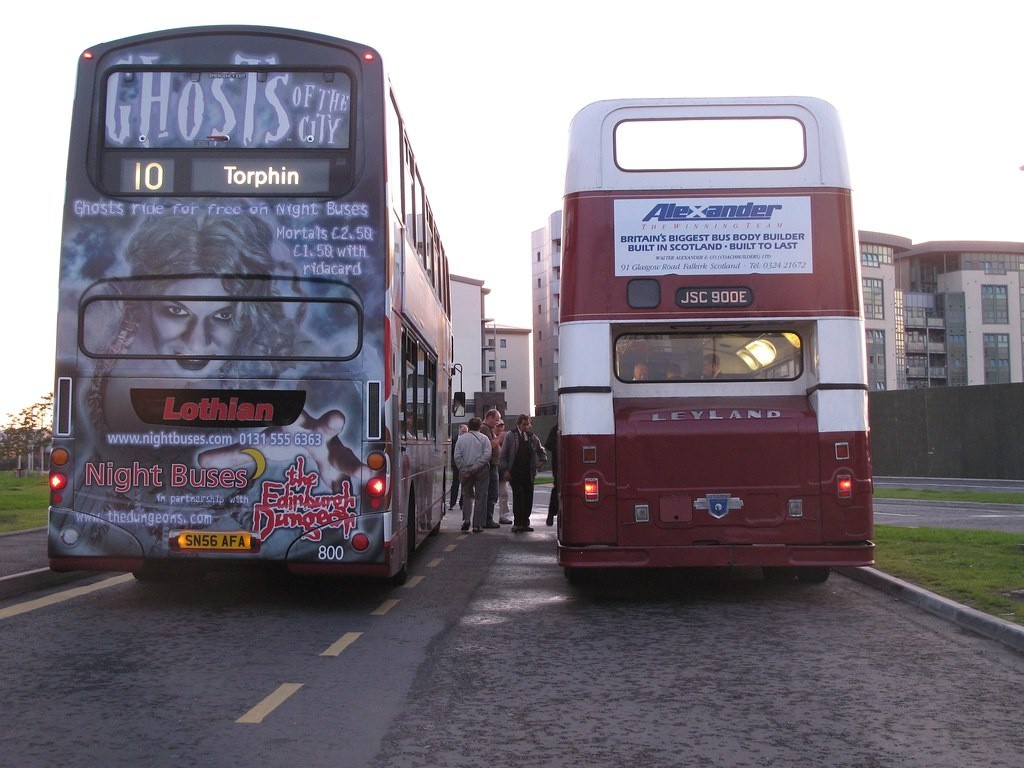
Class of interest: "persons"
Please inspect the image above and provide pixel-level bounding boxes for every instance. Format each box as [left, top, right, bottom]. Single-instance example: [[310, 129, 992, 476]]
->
[[454, 418, 492, 532], [447, 424, 468, 510], [481, 409, 506, 529], [62, 196, 372, 493], [498, 414, 547, 532], [632, 362, 650, 381], [494, 419, 513, 524], [703, 353, 721, 379], [666, 364, 682, 380], [454, 396, 464, 416]]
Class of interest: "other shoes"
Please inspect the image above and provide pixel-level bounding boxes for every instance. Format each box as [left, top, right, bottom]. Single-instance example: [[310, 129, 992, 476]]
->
[[499, 518, 512, 524], [447, 504, 453, 509], [546, 512, 554, 526], [487, 522, 500, 528], [462, 519, 470, 530], [473, 527, 484, 532], [511, 525, 534, 532]]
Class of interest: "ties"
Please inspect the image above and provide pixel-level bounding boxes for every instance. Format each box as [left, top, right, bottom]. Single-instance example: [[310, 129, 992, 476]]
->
[[521, 432, 525, 441]]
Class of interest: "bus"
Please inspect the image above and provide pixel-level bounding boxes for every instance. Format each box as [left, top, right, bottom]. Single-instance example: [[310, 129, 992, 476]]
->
[[553, 95, 879, 593], [43, 20, 468, 589]]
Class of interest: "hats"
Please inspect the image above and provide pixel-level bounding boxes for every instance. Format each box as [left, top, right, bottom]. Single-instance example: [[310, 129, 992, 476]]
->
[[495, 418, 504, 426]]
